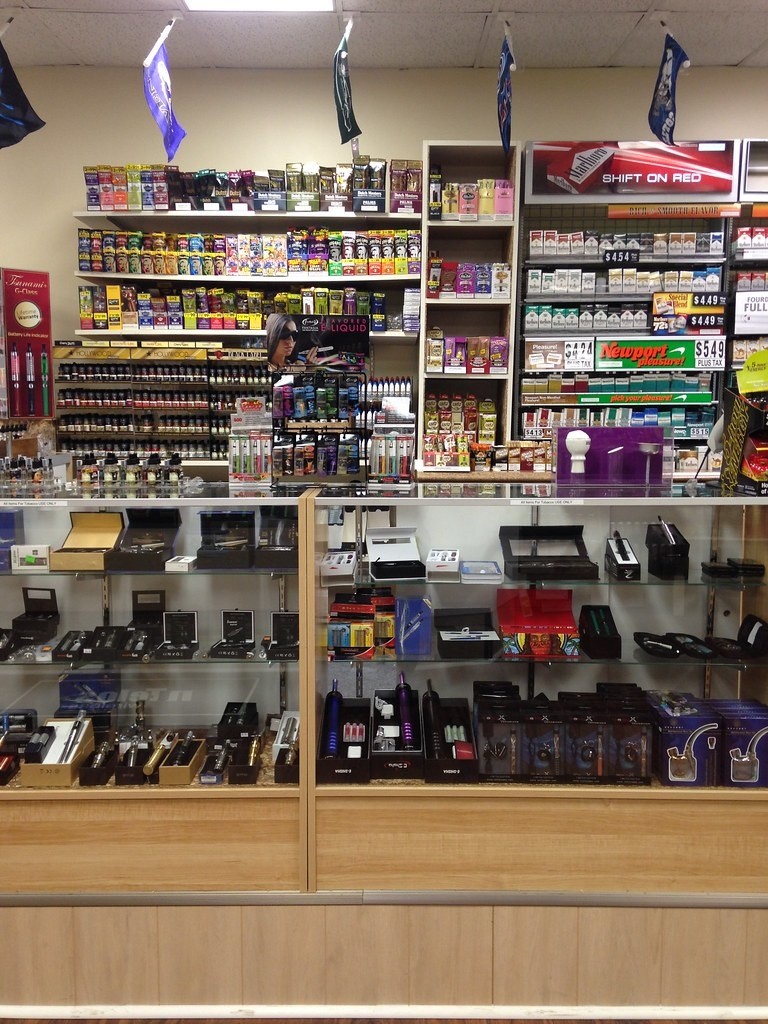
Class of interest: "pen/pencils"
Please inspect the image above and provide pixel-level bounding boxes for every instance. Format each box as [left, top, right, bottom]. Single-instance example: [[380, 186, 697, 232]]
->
[[371, 436, 414, 474], [228, 434, 272, 474], [11, 341, 49, 417]]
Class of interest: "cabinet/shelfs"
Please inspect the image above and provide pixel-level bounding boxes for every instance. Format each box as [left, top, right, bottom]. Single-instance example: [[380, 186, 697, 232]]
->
[[517, 256, 727, 479], [55, 341, 417, 480], [0, 483, 309, 897], [421, 140, 520, 497], [308, 484, 767, 899], [729, 247, 768, 427], [72, 210, 419, 337]]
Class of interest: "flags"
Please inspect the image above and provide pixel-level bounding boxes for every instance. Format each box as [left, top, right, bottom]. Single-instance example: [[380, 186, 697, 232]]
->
[[333, 39, 362, 145], [498, 38, 512, 153], [142, 42, 187, 163], [0, 40, 46, 150], [648, 34, 689, 147]]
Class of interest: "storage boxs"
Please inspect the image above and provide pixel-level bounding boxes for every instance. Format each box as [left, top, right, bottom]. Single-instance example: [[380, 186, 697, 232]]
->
[[1, 506, 767, 794], [80, 164, 768, 473]]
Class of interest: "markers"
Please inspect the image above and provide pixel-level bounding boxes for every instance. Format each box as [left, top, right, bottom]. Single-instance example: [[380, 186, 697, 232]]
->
[[359, 375, 413, 461]]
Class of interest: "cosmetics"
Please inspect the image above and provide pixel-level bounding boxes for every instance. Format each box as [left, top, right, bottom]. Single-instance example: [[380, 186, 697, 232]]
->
[[0, 455, 56, 486], [81, 451, 184, 485]]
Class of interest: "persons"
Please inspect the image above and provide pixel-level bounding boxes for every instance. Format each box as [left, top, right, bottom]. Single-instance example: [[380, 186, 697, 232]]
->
[[265, 314, 319, 373]]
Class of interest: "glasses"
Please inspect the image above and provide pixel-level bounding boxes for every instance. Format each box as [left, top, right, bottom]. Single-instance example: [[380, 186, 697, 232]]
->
[[278, 327, 298, 341]]
